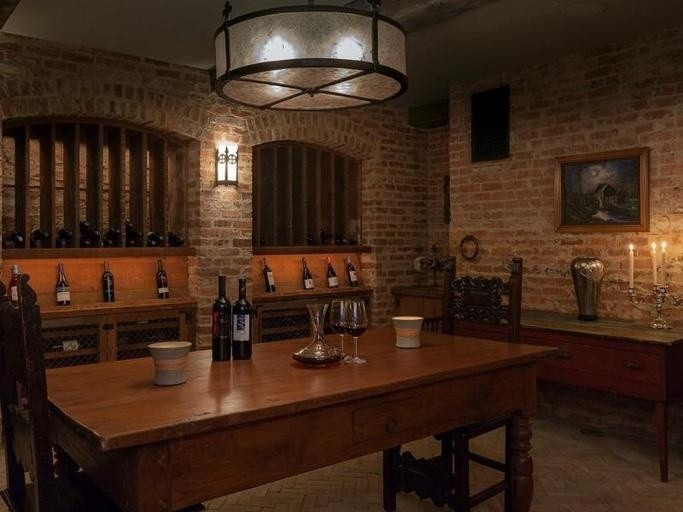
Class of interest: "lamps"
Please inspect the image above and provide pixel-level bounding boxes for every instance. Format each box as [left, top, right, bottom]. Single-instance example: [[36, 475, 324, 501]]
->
[[216, 141, 239, 185], [213, 0, 409, 111]]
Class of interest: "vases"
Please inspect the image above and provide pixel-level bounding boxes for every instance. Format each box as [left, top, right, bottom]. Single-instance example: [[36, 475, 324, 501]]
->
[[570, 257, 604, 321]]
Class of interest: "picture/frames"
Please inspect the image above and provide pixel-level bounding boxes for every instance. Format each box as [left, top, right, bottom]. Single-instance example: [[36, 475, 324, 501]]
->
[[553, 146, 650, 233]]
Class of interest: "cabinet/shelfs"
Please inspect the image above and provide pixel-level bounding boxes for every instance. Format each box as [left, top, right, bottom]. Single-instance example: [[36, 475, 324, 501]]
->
[[253, 286, 373, 343], [16, 293, 197, 409], [391, 284, 444, 334]]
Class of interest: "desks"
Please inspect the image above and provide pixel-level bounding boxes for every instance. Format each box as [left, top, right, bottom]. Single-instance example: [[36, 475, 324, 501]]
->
[[45, 324, 560, 512], [455, 310, 682, 482]]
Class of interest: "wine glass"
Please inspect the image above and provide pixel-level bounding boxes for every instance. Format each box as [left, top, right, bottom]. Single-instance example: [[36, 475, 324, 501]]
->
[[329, 298, 351, 364], [345, 299, 369, 366]]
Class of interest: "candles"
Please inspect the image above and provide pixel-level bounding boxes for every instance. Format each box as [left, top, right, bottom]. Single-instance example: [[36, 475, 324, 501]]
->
[[628, 242, 668, 288]]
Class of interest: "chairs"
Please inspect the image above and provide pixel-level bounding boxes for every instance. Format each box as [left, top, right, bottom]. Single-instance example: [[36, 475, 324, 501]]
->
[[383, 258, 522, 512], [0, 274, 205, 512]]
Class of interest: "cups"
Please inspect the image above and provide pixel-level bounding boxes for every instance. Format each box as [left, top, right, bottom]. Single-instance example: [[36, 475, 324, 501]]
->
[[147, 341, 191, 385], [390, 315, 425, 349]]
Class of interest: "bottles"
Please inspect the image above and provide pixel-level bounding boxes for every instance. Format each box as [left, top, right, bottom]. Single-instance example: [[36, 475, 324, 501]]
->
[[6, 264, 21, 308], [210, 274, 232, 362], [300, 257, 314, 290], [260, 258, 277, 293], [101, 259, 115, 302], [155, 257, 169, 300], [253, 227, 361, 247], [54, 263, 71, 307], [205, 363, 232, 415], [230, 277, 254, 360], [2, 215, 189, 247], [344, 257, 360, 289], [323, 256, 339, 288]]
